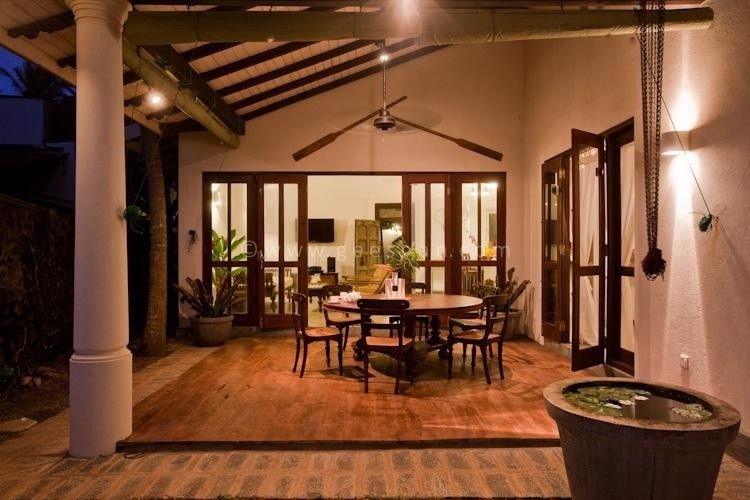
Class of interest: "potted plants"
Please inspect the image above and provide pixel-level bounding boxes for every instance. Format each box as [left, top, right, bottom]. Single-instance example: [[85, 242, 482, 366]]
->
[[472, 266, 523, 341], [174, 273, 242, 347]]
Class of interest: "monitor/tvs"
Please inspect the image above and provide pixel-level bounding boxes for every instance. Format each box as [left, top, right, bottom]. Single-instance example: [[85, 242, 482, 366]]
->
[[308, 218, 335, 244]]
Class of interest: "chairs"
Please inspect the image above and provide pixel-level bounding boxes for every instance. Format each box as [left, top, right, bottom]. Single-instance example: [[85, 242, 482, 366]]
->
[[355, 268, 392, 293], [288, 278, 512, 395]]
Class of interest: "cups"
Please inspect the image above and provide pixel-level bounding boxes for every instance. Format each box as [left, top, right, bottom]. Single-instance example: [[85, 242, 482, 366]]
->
[[327, 291, 361, 304]]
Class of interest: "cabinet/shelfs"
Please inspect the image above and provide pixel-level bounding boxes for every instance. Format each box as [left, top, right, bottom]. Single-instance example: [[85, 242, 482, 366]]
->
[[319, 273, 339, 285]]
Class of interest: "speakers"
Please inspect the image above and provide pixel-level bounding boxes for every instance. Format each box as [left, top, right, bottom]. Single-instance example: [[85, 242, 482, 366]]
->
[[326, 256, 335, 272]]
